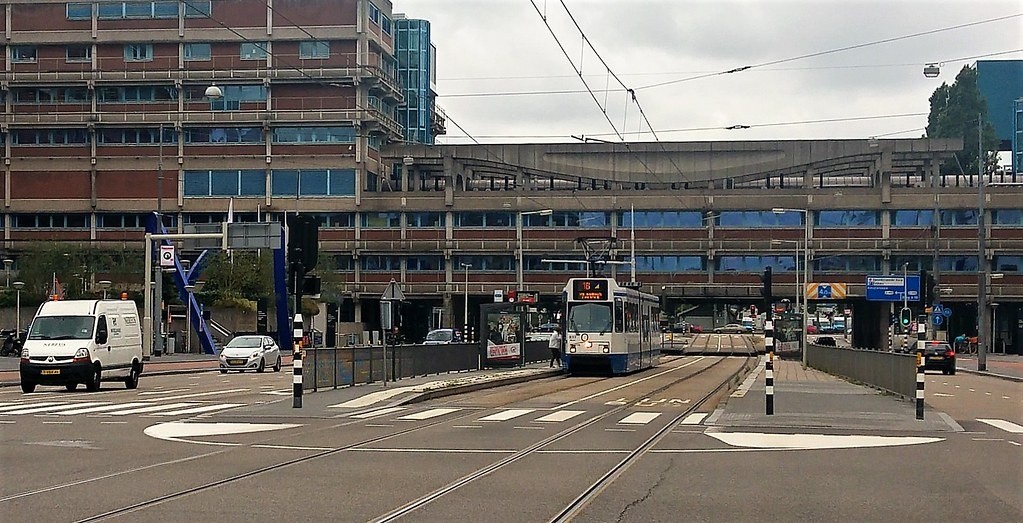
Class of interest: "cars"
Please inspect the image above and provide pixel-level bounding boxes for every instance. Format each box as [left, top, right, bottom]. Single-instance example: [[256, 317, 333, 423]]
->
[[712, 324, 747, 334], [731, 308, 766, 332], [662, 322, 703, 334], [422, 329, 459, 344], [806, 319, 845, 334], [813, 336, 837, 346], [903, 340, 956, 376], [219, 334, 282, 373]]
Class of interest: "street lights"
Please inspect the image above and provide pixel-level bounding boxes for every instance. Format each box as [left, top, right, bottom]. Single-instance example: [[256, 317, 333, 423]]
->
[[184, 285, 197, 354], [990, 302, 999, 354], [772, 208, 808, 370], [99, 281, 112, 300], [180, 260, 190, 277], [519, 209, 553, 291], [149, 282, 157, 355], [462, 262, 472, 341], [12, 282, 25, 359], [901, 261, 909, 355], [3, 259, 14, 288]]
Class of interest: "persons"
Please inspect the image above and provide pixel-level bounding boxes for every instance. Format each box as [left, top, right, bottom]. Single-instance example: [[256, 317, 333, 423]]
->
[[549, 327, 562, 368]]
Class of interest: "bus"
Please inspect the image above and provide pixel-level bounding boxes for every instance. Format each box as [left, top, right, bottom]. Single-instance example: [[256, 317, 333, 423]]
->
[[562, 236, 663, 376]]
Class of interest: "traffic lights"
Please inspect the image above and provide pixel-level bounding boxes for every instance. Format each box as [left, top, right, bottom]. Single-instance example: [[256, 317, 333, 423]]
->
[[900, 308, 912, 326], [751, 305, 755, 314], [761, 267, 773, 321]]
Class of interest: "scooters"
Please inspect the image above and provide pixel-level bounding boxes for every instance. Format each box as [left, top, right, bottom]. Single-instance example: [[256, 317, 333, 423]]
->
[[1, 331, 21, 357]]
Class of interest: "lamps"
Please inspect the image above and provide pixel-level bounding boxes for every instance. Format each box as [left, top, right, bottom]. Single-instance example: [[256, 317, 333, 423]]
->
[[923, 63, 940, 77], [205, 82, 225, 99]]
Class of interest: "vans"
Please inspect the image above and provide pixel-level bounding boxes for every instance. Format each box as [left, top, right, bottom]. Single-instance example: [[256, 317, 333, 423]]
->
[[19, 292, 144, 393]]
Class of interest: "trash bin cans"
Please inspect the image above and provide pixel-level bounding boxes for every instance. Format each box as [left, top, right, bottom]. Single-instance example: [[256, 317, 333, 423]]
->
[[339, 334, 346, 347], [167, 338, 175, 354], [372, 331, 379, 345], [363, 331, 369, 346]]
